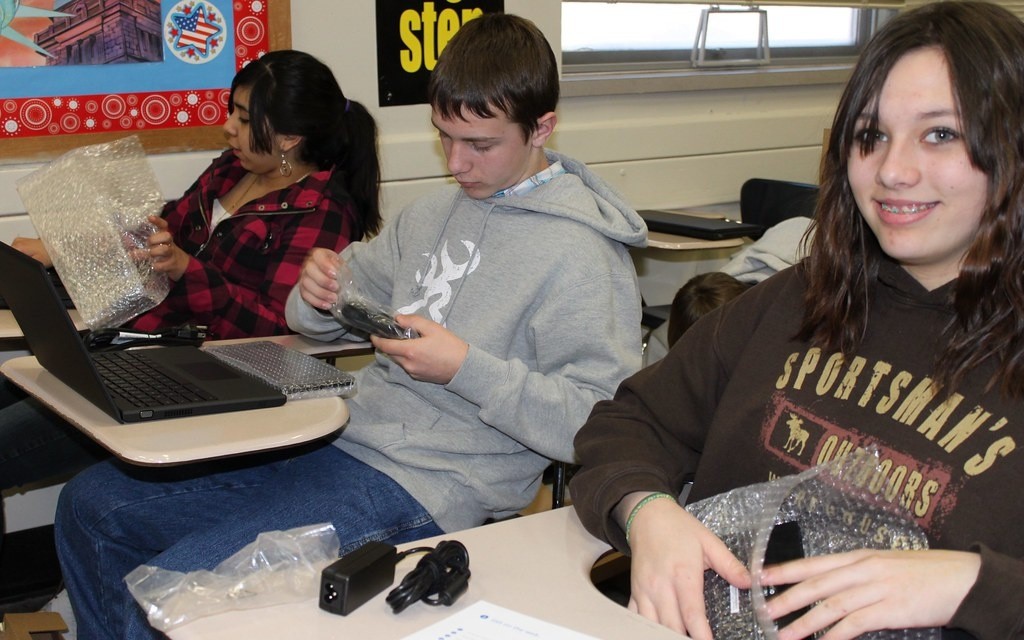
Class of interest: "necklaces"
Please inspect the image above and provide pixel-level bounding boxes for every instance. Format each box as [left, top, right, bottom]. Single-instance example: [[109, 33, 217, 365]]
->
[[216, 175, 259, 224]]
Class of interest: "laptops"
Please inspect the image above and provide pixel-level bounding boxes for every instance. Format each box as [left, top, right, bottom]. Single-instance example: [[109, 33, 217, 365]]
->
[[1, 241, 289, 425]]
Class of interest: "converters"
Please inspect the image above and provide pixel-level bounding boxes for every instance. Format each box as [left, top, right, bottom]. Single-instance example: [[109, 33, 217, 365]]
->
[[318, 540, 397, 618]]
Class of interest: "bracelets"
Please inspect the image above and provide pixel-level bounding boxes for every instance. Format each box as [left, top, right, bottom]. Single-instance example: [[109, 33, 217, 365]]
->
[[626, 493, 677, 545]]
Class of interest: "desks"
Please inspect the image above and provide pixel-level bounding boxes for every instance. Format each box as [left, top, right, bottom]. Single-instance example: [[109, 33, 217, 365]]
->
[[624, 208, 743, 251], [0, 310, 91, 336], [2, 353, 349, 465], [154, 505, 702, 640]]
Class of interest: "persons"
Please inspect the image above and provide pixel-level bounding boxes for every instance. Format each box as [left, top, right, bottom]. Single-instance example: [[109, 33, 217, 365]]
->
[[54, 15, 645, 640], [0, 50, 378, 621], [570, 0, 1024, 640]]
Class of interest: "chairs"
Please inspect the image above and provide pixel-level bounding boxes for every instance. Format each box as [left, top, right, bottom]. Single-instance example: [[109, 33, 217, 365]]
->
[[740, 177, 820, 242]]
